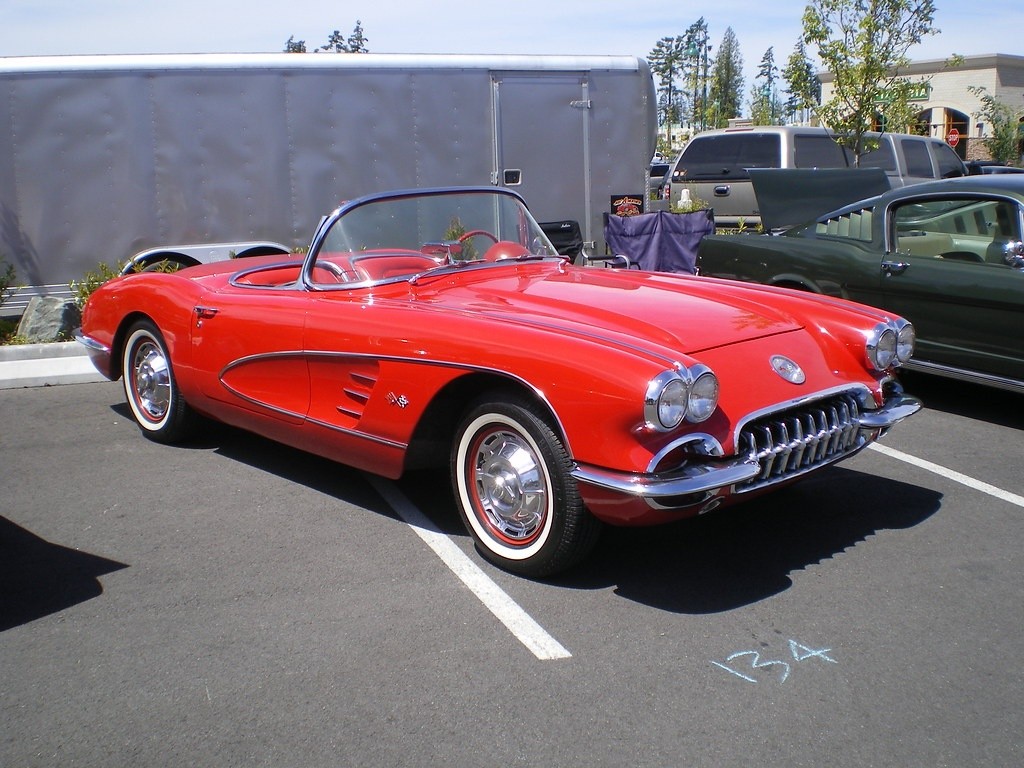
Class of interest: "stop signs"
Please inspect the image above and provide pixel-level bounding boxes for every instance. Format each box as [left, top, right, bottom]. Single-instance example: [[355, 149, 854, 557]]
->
[[948, 129, 959, 148]]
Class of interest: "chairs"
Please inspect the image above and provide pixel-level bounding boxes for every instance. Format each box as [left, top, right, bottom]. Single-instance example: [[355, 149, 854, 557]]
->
[[515, 220, 631, 270], [602, 206, 715, 274]]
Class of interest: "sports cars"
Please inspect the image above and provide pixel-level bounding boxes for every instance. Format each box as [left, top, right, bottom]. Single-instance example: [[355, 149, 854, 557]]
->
[[67, 185, 925, 581]]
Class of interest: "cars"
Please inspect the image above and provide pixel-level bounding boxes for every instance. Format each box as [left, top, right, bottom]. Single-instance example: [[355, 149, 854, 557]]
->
[[651, 151, 665, 164], [658, 130, 1024, 403]]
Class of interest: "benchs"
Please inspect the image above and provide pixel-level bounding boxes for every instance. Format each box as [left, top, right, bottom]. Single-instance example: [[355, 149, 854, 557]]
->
[[173, 251, 355, 289], [897, 233, 954, 257], [223, 248, 439, 290]]
